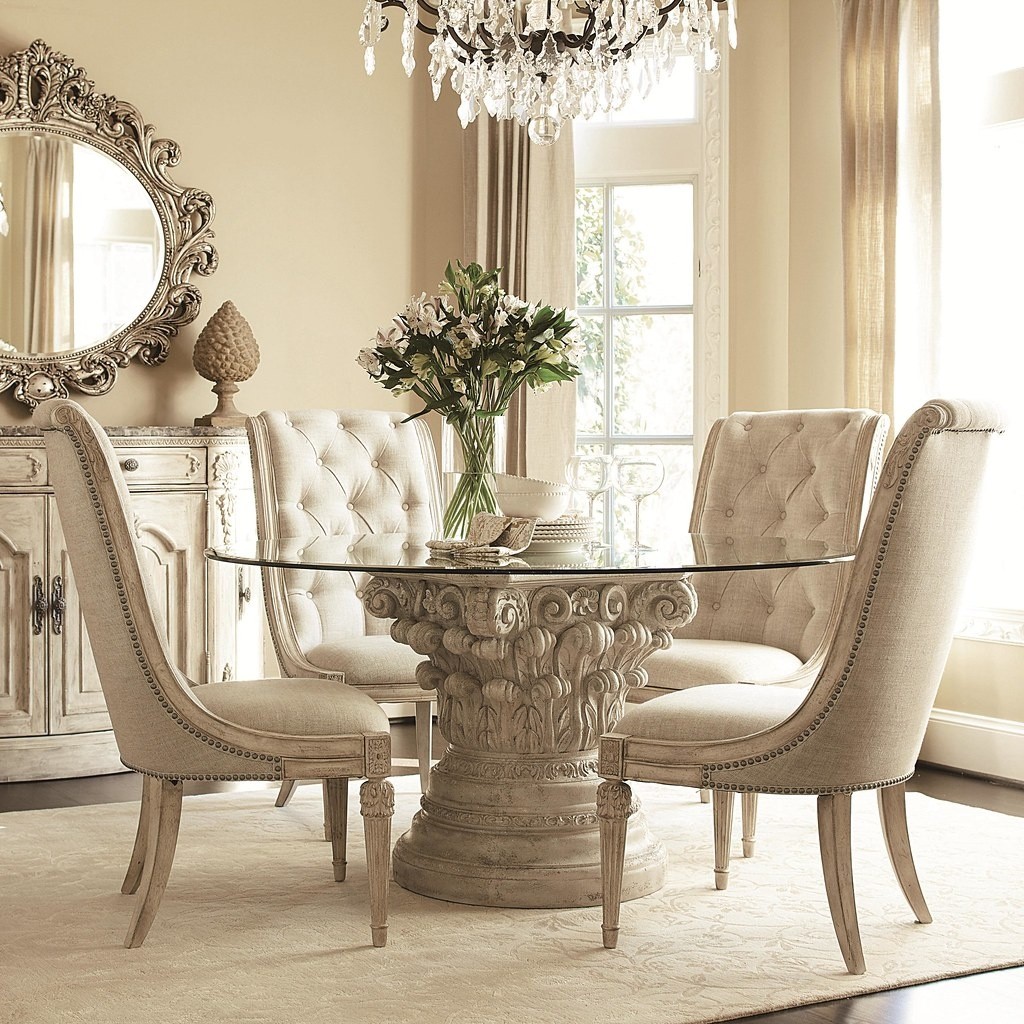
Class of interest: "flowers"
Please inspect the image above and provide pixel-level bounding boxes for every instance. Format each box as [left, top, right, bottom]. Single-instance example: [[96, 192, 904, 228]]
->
[[351, 258, 583, 538]]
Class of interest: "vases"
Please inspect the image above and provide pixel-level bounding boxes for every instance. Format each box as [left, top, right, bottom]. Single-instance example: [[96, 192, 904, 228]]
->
[[432, 411, 505, 540]]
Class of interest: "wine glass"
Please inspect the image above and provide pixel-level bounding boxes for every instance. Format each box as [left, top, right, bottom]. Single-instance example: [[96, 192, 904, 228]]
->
[[566, 454, 665, 568]]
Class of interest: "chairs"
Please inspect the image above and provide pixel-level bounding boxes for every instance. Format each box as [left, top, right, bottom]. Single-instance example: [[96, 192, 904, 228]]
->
[[599, 394, 1011, 975], [249, 409, 448, 837], [628, 407, 885, 857], [35, 399, 396, 950]]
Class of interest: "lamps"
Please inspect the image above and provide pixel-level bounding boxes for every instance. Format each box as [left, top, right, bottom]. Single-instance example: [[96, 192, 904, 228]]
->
[[359, 1, 738, 151]]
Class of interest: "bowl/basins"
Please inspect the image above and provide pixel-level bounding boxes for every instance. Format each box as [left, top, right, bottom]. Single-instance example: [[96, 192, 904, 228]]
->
[[495, 472, 570, 493], [495, 491, 571, 522]]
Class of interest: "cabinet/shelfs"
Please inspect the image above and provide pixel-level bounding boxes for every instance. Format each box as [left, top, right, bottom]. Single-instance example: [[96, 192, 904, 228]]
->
[[205, 538, 855, 909]]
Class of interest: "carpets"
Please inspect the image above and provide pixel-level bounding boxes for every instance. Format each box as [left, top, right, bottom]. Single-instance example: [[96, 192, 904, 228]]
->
[[0, 768, 1024, 1024]]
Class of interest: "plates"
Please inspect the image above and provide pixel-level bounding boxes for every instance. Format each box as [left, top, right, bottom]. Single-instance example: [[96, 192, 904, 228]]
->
[[528, 515, 593, 552]]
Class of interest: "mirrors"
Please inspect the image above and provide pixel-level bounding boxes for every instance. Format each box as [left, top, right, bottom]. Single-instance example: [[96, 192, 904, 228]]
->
[[0, 40, 221, 413]]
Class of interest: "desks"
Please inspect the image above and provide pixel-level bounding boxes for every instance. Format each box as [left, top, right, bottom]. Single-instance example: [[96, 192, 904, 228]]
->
[[0, 428, 236, 811]]
